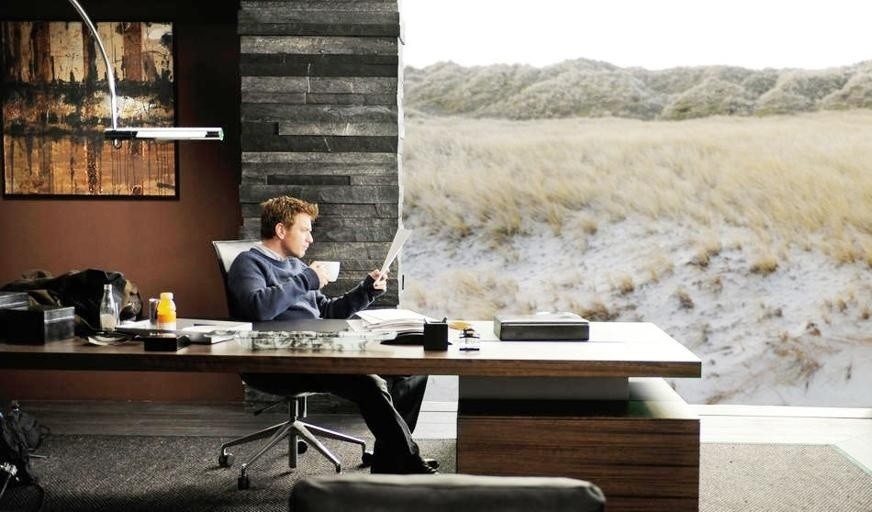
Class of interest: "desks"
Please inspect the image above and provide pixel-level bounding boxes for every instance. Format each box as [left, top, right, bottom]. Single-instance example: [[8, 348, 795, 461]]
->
[[0, 315, 703, 512]]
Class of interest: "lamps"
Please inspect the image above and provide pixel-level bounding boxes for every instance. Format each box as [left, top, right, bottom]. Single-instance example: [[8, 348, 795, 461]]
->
[[70, 0, 225, 149]]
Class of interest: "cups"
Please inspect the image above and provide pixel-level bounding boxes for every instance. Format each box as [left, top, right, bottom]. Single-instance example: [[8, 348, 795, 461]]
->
[[317, 261, 340, 282]]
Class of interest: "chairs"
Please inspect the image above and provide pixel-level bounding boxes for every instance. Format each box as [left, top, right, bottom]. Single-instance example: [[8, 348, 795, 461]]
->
[[287, 473, 609, 512], [209, 238, 375, 492]]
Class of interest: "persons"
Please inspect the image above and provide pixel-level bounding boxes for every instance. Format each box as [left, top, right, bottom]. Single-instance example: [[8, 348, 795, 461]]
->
[[227, 195, 440, 474]]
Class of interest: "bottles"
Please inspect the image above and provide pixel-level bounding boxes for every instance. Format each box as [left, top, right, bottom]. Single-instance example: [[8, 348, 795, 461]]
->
[[157, 291, 176, 332], [99, 283, 120, 330], [458, 328, 480, 350]]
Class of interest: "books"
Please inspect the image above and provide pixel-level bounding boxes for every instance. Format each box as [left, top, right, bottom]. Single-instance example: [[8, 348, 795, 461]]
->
[[493, 314, 589, 340], [347, 309, 437, 333]]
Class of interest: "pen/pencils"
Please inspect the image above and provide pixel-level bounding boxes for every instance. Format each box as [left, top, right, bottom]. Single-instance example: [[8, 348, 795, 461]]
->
[[194, 323, 216, 326]]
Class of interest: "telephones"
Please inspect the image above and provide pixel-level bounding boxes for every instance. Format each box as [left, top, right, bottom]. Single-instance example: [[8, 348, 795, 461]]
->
[[397, 330, 426, 345]]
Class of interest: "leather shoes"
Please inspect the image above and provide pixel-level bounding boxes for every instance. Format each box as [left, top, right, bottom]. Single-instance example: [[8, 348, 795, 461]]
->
[[371, 454, 440, 475]]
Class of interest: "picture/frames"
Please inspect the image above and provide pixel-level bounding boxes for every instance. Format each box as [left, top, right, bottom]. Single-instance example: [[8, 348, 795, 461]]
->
[[0, 14, 181, 202]]
